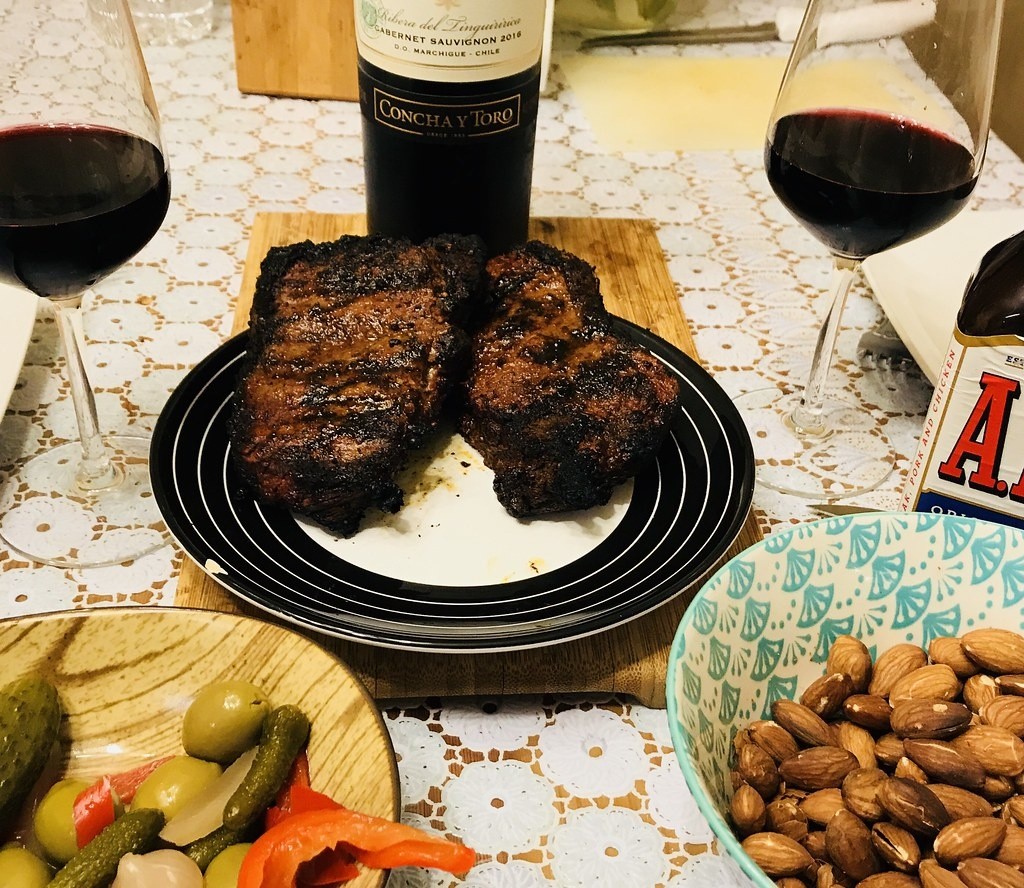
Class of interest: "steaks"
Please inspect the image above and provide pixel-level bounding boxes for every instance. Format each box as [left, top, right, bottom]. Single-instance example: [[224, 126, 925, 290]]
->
[[239, 232, 683, 538]]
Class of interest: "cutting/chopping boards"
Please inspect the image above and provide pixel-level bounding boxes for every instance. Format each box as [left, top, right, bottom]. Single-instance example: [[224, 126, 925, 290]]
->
[[172, 212, 766, 708]]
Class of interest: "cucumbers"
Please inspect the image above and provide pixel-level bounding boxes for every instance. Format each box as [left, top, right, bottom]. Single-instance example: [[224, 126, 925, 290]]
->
[[0, 671, 313, 888]]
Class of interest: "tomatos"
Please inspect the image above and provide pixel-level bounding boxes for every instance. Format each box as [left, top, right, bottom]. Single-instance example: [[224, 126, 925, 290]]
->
[[239, 785, 479, 888]]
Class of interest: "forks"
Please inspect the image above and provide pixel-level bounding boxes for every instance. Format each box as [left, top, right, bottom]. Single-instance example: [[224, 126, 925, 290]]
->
[[855, 314, 924, 406]]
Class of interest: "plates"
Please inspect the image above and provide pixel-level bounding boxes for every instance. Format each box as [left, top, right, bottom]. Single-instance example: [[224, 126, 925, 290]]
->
[[148, 328, 756, 654], [0, 605, 402, 888]]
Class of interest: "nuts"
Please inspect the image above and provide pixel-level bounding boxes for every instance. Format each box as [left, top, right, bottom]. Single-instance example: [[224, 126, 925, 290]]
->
[[727, 627, 1024, 888]]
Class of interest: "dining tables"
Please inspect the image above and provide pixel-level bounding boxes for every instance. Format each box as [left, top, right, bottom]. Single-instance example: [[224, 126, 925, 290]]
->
[[0, 0, 1024, 888]]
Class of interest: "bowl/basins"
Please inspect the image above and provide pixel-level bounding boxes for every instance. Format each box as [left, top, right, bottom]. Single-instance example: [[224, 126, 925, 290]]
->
[[664, 512, 1024, 888]]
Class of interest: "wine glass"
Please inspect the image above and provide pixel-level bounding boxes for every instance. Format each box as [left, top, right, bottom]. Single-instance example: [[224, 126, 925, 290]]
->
[[729, 0, 1003, 502], [0, 0, 173, 570]]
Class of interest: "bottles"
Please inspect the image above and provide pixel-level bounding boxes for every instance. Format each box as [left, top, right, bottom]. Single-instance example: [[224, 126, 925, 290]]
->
[[354, 1, 548, 247], [898, 229, 1022, 533]]
